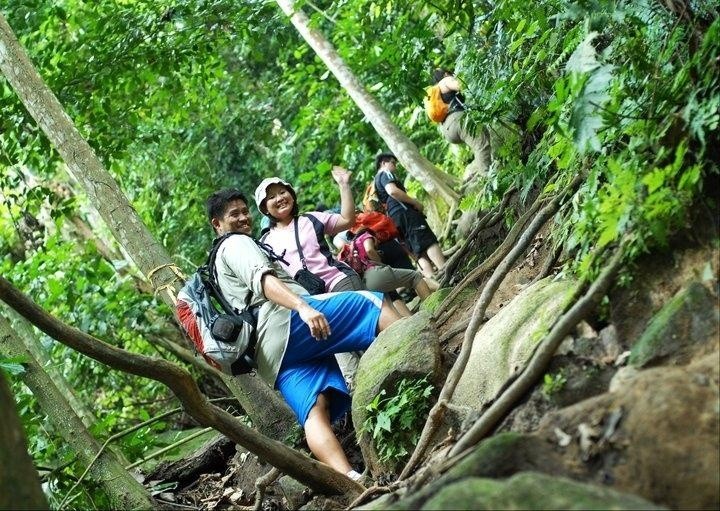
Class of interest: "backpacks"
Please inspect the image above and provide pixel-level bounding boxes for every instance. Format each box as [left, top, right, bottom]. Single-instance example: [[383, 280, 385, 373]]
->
[[339, 242, 368, 274], [361, 175, 390, 215], [175, 231, 290, 376], [423, 85, 463, 122]]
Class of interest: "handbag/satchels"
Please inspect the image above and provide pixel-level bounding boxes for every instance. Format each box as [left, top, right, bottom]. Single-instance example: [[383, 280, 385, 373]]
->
[[293, 269, 325, 295]]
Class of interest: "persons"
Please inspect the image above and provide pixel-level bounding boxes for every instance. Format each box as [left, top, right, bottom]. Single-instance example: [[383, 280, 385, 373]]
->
[[205, 187, 405, 487], [433, 65, 491, 170], [314, 153, 450, 320], [252, 164, 368, 299]]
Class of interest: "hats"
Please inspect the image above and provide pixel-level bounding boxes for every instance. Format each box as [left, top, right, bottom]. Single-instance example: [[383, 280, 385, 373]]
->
[[260, 216, 270, 236], [256, 177, 295, 216]]
